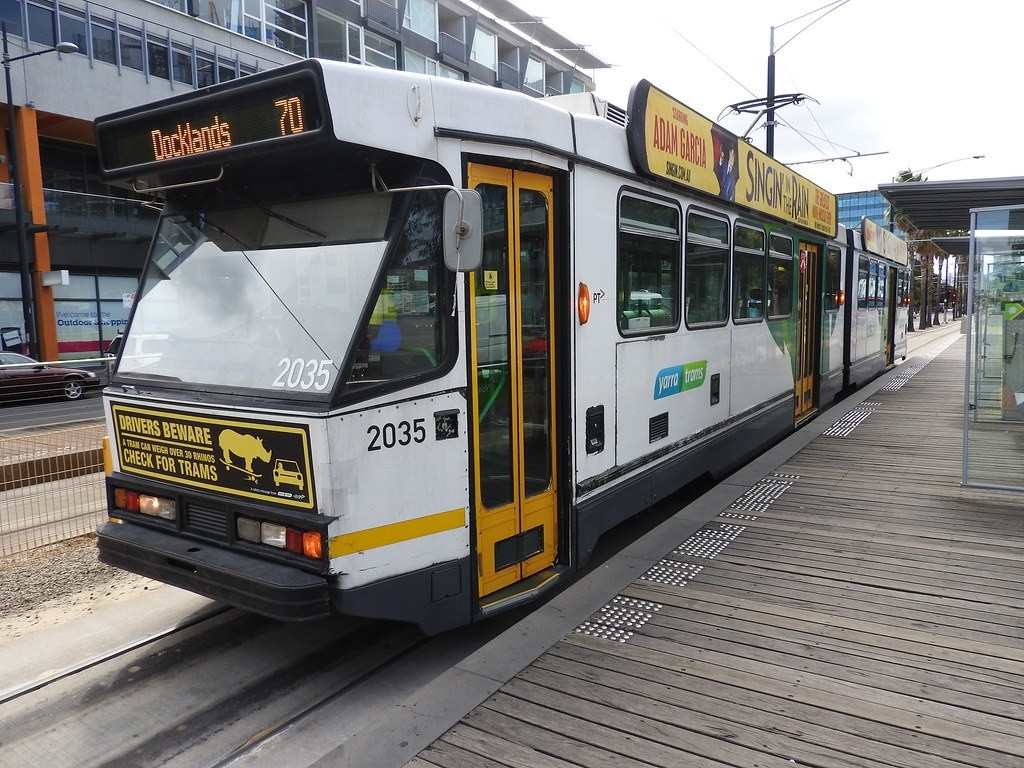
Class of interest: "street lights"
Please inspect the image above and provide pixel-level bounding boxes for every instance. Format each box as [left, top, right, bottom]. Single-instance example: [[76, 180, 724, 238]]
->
[[894, 154, 987, 182], [0, 26, 82, 360]]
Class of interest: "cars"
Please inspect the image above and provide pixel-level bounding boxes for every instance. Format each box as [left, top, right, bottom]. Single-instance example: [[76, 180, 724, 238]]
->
[[0, 352, 102, 403]]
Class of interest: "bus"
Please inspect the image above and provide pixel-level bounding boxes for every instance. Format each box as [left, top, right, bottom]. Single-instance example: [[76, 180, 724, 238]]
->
[[87, 56, 849, 637], [848, 215, 914, 388]]
